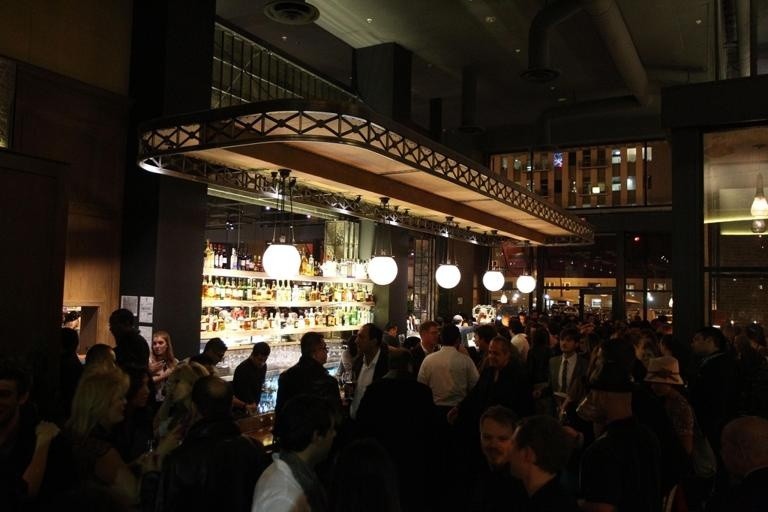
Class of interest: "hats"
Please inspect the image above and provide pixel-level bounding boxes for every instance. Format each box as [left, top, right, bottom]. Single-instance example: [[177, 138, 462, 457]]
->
[[591, 361, 635, 394], [645, 356, 684, 384]]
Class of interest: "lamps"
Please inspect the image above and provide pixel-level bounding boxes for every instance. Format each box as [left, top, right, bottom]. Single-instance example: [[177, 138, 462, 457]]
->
[[435, 216, 463, 288], [752, 219, 766, 234], [261, 167, 304, 283], [483, 225, 504, 293], [366, 196, 399, 286], [750, 171, 768, 218], [515, 235, 537, 296]]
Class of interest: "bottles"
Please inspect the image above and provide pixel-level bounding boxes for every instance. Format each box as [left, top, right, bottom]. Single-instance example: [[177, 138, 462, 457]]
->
[[200, 239, 379, 283], [197, 305, 374, 329], [199, 275, 374, 303]]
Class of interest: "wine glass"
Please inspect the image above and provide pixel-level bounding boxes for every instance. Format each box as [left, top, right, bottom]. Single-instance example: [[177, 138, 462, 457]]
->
[[208, 340, 348, 368]]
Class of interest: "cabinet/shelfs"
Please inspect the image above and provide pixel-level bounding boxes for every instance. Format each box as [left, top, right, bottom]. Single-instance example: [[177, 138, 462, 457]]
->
[[203, 261, 378, 353]]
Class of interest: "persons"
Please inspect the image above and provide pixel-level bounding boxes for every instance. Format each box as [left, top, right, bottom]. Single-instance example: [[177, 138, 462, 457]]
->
[[3, 309, 270, 511], [252, 394, 337, 512], [337, 293, 768, 512], [275, 332, 342, 464]]
